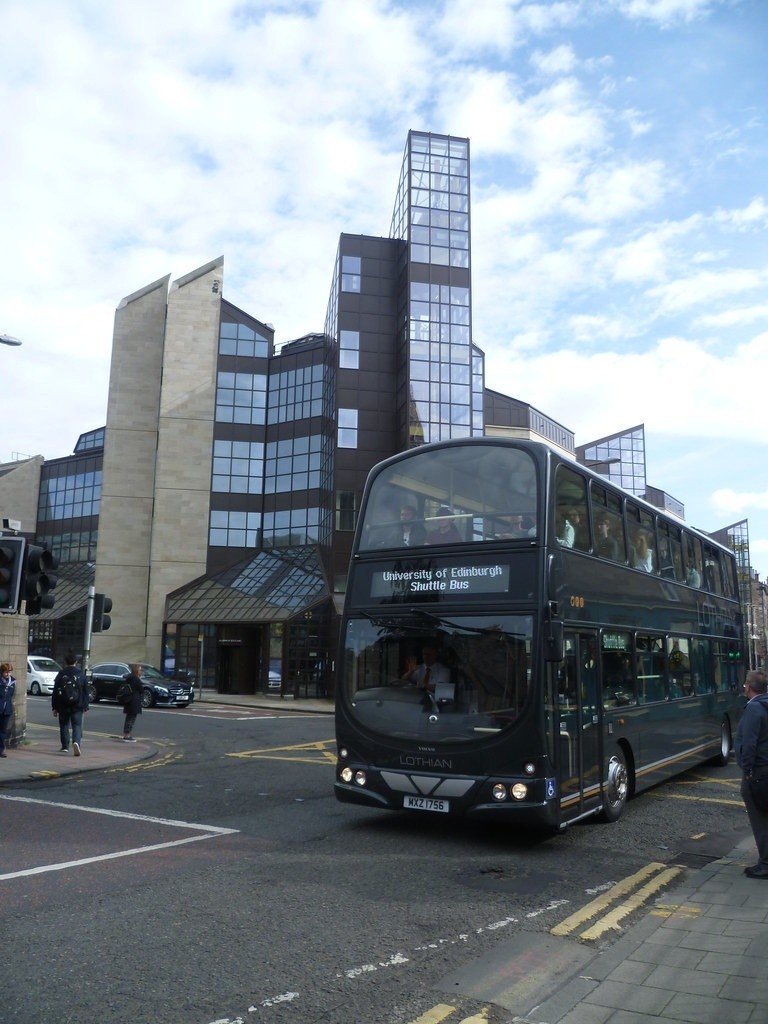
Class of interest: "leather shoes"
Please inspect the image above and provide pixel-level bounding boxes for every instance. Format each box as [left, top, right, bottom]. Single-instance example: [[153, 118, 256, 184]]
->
[[744, 864, 768, 879]]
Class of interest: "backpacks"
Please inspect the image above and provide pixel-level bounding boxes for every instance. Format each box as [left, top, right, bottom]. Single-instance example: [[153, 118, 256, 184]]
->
[[56, 670, 83, 705], [122, 679, 136, 701]]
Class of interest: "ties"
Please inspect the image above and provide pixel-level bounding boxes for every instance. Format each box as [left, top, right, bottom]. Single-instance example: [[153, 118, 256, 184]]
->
[[424, 668, 430, 687]]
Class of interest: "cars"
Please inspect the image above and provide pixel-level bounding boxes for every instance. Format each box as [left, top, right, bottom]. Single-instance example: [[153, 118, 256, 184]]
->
[[268, 671, 281, 688], [27, 656, 64, 696], [164, 645, 175, 673]]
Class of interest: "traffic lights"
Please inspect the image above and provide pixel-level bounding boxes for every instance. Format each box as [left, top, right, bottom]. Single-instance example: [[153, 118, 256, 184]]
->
[[0, 536, 26, 614], [19, 544, 53, 602], [40, 556, 59, 609], [724, 625, 742, 663], [92, 594, 113, 632]]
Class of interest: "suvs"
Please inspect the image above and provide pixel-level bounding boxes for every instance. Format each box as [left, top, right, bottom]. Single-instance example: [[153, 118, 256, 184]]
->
[[87, 662, 194, 709]]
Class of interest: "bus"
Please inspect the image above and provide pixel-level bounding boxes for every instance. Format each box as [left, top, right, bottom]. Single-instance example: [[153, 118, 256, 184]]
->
[[333, 437, 750, 837]]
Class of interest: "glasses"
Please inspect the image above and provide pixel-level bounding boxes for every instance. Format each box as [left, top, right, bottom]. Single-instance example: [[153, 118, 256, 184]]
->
[[742, 682, 750, 689]]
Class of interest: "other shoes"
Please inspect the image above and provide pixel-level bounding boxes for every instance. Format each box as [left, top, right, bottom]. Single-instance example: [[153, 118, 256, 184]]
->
[[1, 751, 7, 757], [60, 747, 69, 752], [123, 736, 137, 742], [72, 742, 81, 756]]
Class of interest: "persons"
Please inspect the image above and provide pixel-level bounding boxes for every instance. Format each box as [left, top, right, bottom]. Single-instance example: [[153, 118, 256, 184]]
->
[[632, 536, 653, 573], [51, 652, 89, 756], [684, 557, 700, 589], [423, 506, 463, 545], [401, 641, 451, 690], [658, 547, 674, 579], [594, 514, 618, 561], [527, 504, 575, 549], [385, 505, 427, 549], [0, 663, 17, 757], [121, 664, 144, 743], [734, 668, 768, 880], [564, 507, 590, 552], [498, 514, 530, 539]]
